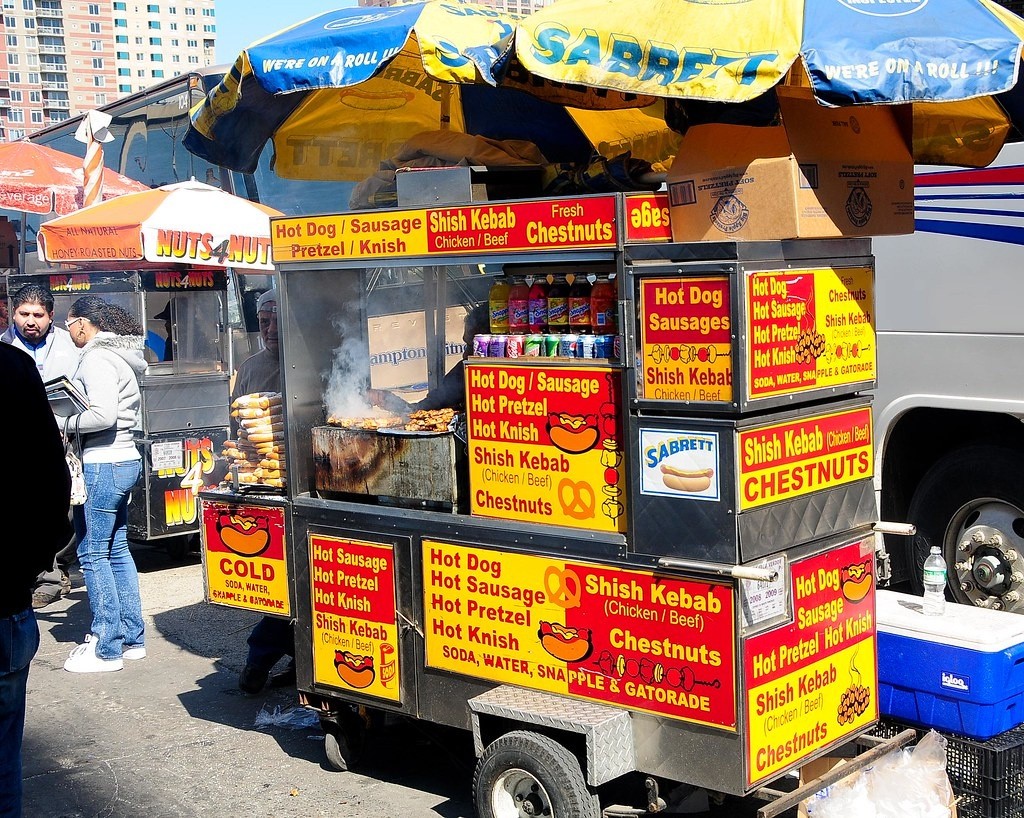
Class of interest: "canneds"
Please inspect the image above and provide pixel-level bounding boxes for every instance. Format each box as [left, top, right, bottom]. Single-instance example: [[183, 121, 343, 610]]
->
[[472, 333, 621, 361]]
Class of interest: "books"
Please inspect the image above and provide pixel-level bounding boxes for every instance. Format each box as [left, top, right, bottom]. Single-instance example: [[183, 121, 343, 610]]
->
[[44, 374, 90, 418]]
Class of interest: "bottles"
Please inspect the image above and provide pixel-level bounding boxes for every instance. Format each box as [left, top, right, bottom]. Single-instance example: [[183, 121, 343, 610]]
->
[[508, 281, 529, 334], [489, 280, 509, 334], [923, 546, 947, 615], [568, 279, 592, 334], [528, 280, 548, 333], [547, 279, 569, 335], [591, 275, 618, 334]]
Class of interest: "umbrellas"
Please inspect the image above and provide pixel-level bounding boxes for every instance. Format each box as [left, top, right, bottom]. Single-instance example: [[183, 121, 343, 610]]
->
[[37, 173, 287, 353], [0, 137, 152, 275], [181, 1, 1024, 180]]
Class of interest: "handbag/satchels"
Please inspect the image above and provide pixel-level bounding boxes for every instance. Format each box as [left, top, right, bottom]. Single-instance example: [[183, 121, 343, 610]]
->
[[59, 414, 89, 506]]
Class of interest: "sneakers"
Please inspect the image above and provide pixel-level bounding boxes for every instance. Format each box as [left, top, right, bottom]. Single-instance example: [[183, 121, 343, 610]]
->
[[121, 647, 147, 660], [64, 633, 123, 672]]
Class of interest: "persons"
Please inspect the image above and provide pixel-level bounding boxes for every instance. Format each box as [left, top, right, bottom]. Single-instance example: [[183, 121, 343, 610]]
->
[[53, 293, 146, 674], [0, 282, 82, 608], [154, 296, 223, 362], [0, 343, 75, 818], [227, 287, 283, 456]]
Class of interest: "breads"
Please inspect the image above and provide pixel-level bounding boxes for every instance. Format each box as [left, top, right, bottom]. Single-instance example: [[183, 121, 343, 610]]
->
[[224, 393, 286, 488]]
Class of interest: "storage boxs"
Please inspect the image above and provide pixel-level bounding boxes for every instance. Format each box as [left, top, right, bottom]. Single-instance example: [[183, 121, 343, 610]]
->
[[666, 96, 915, 242], [858, 712, 1024, 818], [875, 589, 1024, 741]]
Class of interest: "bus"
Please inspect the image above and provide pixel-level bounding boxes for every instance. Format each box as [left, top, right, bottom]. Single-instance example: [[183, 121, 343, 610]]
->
[[2, 62, 1024, 619]]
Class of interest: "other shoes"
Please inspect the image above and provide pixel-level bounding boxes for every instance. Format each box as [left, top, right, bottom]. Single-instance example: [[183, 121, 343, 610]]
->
[[239, 662, 269, 694], [32, 592, 60, 608]]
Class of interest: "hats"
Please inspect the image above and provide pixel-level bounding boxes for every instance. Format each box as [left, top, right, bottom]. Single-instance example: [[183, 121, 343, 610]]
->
[[256, 288, 277, 313], [153, 296, 198, 320]]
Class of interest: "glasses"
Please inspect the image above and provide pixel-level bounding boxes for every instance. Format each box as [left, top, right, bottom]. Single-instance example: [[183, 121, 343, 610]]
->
[[65, 317, 90, 330]]
